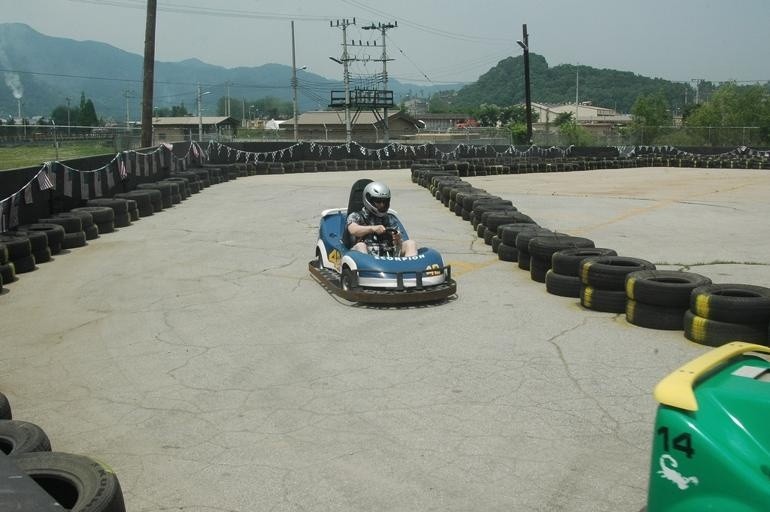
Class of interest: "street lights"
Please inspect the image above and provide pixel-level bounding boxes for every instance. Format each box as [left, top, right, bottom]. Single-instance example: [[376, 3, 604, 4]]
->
[[197, 81, 212, 142], [516, 23, 532, 145], [290, 20, 307, 140]]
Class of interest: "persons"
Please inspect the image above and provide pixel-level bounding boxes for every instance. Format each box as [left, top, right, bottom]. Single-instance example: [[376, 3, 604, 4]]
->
[[346, 182, 418, 257]]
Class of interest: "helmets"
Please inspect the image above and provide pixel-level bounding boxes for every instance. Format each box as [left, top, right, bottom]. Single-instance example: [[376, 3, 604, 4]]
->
[[362, 181, 391, 218]]
